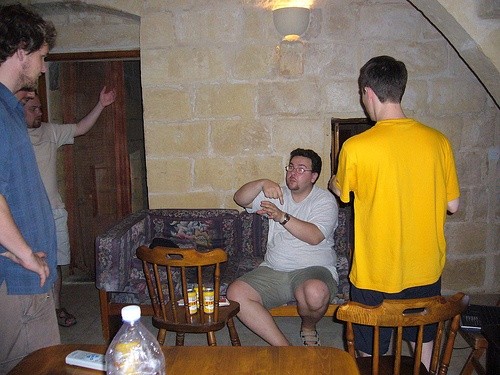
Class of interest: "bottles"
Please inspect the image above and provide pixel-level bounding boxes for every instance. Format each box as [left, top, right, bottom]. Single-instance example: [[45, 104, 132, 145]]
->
[[104, 305, 166, 375]]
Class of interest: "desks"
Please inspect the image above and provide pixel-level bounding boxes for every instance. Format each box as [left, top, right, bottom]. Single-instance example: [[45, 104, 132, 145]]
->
[[7, 344, 361, 375]]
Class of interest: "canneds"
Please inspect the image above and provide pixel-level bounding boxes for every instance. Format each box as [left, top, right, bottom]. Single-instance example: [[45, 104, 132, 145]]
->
[[202, 288, 214, 314], [193, 285, 205, 308], [187, 288, 197, 315], [115, 336, 140, 375]]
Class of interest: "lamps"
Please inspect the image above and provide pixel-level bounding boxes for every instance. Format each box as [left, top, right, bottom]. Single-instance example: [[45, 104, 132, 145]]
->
[[272, 7, 310, 37]]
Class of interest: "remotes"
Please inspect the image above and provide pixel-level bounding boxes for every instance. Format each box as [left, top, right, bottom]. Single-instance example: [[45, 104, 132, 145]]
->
[[65, 350, 107, 371]]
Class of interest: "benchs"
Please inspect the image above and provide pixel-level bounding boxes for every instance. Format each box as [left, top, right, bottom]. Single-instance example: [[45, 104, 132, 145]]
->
[[96, 207, 354, 343]]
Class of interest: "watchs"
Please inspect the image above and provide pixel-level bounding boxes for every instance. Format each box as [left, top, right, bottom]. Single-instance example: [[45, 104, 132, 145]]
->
[[281, 213, 290, 225]]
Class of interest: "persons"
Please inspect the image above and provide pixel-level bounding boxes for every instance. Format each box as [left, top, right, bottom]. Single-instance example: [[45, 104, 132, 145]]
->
[[0, 5, 61, 375], [226, 148, 339, 346], [14, 85, 117, 327], [328, 55, 460, 375]]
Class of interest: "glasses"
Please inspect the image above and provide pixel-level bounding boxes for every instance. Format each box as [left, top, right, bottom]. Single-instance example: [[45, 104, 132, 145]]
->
[[285, 164, 312, 175]]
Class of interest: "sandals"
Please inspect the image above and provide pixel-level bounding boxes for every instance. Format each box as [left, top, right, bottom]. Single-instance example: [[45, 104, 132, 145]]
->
[[58, 306, 77, 327]]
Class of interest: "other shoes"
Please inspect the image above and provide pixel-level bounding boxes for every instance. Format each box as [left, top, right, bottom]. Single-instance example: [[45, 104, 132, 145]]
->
[[299, 327, 321, 346]]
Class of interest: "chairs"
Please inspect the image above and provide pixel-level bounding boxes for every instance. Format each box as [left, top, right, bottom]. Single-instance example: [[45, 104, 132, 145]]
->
[[458, 299, 500, 375], [136, 245, 241, 346], [337, 291, 469, 375]]
